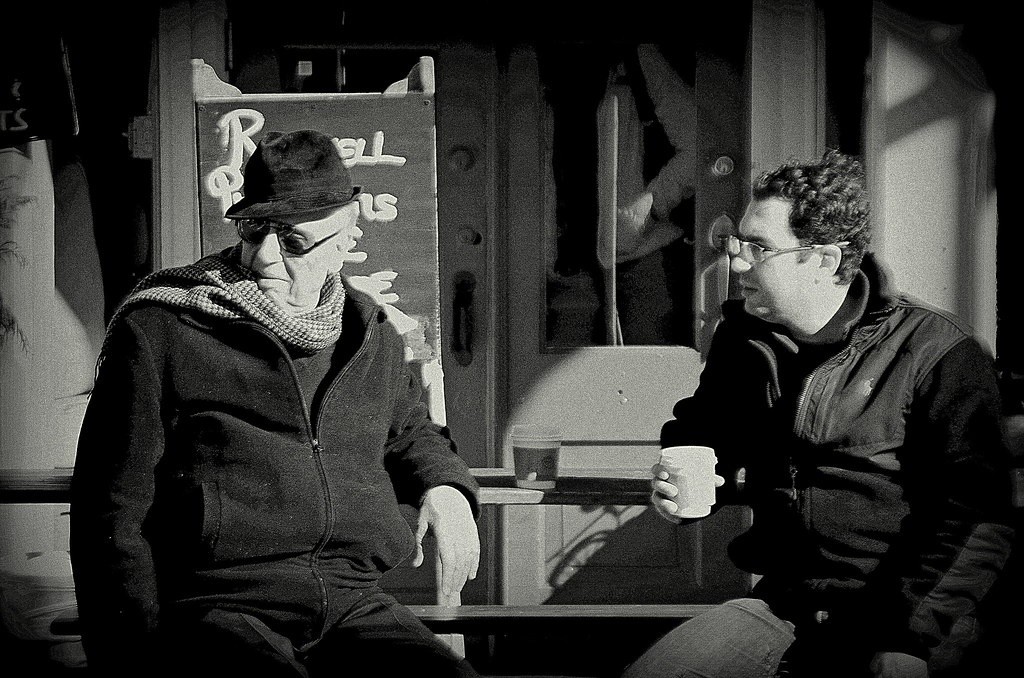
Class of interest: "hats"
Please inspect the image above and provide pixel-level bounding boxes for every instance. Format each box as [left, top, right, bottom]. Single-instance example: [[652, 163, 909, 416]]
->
[[225, 129, 363, 218]]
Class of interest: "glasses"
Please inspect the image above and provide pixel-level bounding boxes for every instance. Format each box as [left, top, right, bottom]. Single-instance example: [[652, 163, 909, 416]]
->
[[721, 234, 850, 265], [235, 217, 343, 255]]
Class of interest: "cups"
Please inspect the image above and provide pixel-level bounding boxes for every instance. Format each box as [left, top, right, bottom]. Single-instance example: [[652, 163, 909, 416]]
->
[[510, 425, 563, 490], [658, 445, 719, 519]]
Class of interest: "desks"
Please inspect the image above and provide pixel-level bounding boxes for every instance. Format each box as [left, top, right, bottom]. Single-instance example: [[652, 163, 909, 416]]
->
[[0, 468, 751, 678]]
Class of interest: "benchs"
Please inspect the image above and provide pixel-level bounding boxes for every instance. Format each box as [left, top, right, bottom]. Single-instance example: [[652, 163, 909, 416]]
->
[[50, 604, 723, 634]]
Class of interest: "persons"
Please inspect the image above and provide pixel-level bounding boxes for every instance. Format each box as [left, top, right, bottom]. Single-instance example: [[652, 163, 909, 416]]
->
[[620, 150, 1019, 678], [69, 130, 481, 678]]
